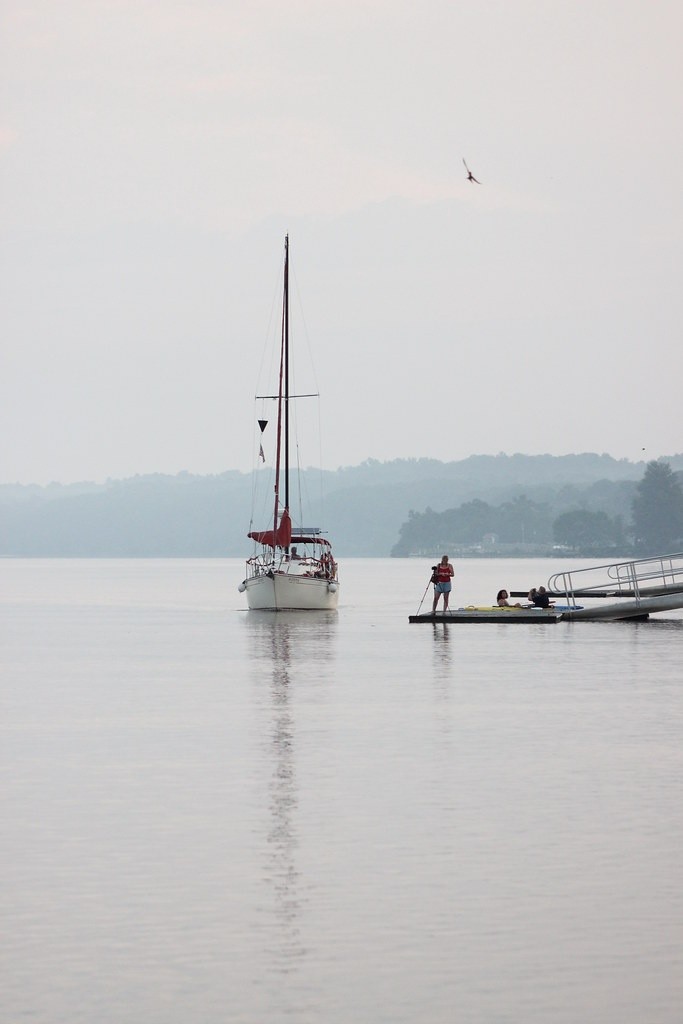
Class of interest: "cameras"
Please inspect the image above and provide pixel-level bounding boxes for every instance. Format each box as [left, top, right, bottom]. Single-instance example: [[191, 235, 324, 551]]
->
[[432, 566, 437, 570], [415, 570, 453, 621]]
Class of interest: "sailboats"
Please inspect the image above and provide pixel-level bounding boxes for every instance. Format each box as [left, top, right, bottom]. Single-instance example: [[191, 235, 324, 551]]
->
[[238, 238, 340, 612]]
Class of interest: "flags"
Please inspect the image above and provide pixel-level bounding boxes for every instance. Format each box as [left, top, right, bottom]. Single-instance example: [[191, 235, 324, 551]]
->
[[259, 445, 265, 462]]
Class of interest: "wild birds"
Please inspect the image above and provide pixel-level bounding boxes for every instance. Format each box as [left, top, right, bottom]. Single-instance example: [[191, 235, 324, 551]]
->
[[462, 159, 483, 185]]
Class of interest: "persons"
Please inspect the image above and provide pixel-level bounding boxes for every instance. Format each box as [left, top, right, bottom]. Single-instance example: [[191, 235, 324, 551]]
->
[[431, 555, 454, 615], [528, 586, 548, 608], [496, 589, 509, 607], [290, 547, 301, 560]]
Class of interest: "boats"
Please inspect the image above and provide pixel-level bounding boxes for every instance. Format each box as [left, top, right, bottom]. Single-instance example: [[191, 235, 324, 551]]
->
[[409, 606, 584, 624]]
